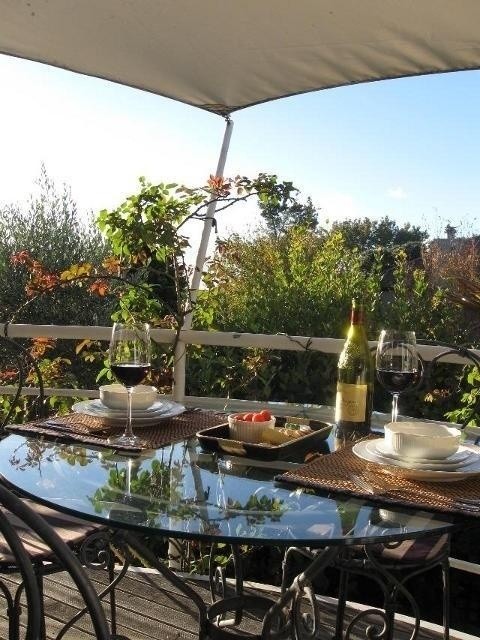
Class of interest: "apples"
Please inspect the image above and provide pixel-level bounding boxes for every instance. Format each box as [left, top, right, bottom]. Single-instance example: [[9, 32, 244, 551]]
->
[[236, 409, 271, 423]]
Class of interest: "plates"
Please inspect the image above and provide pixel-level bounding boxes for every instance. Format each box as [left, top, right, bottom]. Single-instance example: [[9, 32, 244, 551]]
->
[[351, 436, 480, 483], [70, 398, 187, 428]]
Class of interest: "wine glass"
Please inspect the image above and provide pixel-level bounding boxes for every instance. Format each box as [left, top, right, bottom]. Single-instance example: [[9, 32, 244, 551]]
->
[[106, 322, 152, 448], [375, 328, 418, 424], [106, 454, 151, 527]]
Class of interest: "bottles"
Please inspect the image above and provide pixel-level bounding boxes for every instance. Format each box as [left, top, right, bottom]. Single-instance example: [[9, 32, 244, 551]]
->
[[334, 296, 376, 439]]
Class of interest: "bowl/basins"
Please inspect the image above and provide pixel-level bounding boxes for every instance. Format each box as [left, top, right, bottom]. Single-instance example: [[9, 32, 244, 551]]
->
[[383, 421, 462, 461], [227, 410, 276, 444], [99, 383, 158, 411]]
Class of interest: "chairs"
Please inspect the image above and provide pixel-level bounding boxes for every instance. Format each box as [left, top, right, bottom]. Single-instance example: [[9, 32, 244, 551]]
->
[[280, 336, 479, 640], [0, 275, 184, 640]]
